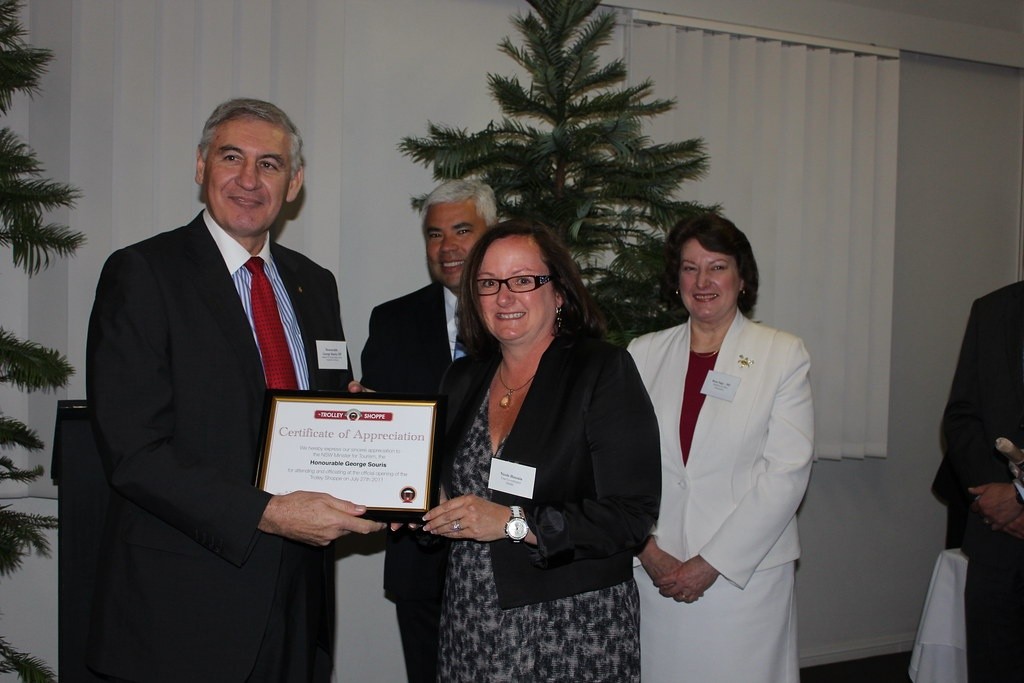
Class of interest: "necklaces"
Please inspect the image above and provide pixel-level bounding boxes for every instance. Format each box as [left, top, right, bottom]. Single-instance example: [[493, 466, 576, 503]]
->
[[499, 362, 535, 408]]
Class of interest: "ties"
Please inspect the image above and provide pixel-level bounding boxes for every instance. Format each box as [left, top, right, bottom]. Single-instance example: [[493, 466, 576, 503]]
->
[[246, 257, 301, 392], [454, 335, 468, 360]]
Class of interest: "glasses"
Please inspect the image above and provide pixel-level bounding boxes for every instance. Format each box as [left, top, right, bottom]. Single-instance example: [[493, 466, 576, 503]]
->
[[475, 275, 552, 295]]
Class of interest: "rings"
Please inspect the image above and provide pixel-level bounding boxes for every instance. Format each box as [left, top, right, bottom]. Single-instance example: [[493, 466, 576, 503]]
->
[[451, 520, 460, 532], [985, 520, 989, 524]]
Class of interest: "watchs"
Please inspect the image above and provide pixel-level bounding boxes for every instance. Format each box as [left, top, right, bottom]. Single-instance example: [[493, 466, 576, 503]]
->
[[504, 505, 529, 544]]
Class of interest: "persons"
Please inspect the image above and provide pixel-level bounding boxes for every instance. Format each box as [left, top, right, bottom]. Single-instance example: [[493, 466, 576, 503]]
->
[[626, 211, 813, 683], [940, 279, 1024, 683], [390, 216, 662, 683], [85, 97, 388, 683], [349, 177, 499, 683]]
